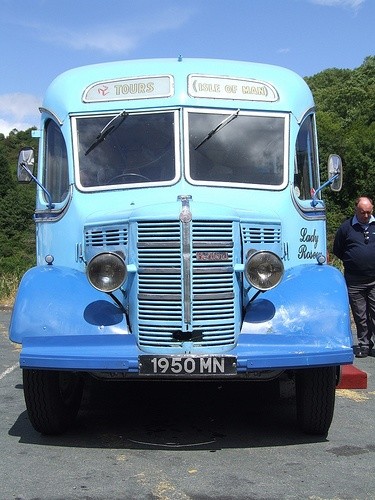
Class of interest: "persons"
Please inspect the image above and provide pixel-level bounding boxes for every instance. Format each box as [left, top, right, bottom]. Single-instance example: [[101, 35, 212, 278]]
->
[[333, 196, 375, 357]]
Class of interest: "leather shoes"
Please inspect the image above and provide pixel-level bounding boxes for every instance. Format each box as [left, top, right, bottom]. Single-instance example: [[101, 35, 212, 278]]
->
[[370, 349, 375, 356], [355, 347, 369, 357]]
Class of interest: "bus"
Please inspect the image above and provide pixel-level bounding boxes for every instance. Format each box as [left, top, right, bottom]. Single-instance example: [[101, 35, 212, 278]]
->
[[12, 54, 353, 435]]
